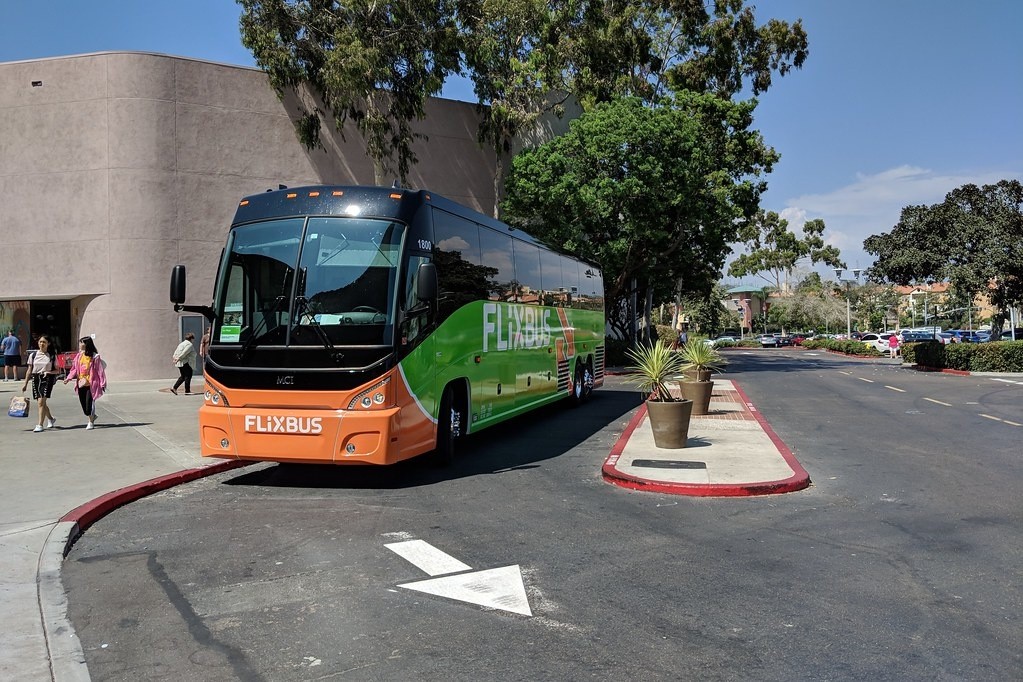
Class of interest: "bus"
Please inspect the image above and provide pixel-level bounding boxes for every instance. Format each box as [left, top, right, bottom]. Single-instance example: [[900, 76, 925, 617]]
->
[[170, 182, 608, 469]]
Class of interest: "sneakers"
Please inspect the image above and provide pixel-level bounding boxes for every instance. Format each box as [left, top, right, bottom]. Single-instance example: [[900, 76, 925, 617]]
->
[[33, 424, 44, 432], [47, 416, 56, 427], [94, 414, 98, 420], [86, 421, 94, 429]]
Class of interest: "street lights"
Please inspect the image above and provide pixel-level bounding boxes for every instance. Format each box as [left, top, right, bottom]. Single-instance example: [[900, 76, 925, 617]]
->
[[832, 268, 863, 341], [732, 298, 752, 341], [875, 305, 892, 334]]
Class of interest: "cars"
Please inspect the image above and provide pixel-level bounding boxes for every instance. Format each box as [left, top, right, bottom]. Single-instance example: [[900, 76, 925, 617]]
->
[[694, 324, 1023, 353]]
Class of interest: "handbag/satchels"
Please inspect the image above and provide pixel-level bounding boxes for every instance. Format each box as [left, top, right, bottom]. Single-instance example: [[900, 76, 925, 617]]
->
[[897, 343, 901, 346], [8, 391, 32, 417], [78, 377, 89, 388], [902, 336, 906, 342]]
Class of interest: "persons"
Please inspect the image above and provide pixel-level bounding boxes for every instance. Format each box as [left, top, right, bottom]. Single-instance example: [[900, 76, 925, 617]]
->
[[170, 333, 197, 395], [678, 329, 687, 348], [63, 336, 107, 430], [200, 327, 211, 369], [889, 332, 905, 358], [950, 334, 970, 343], [0, 328, 22, 382], [22, 331, 60, 432]]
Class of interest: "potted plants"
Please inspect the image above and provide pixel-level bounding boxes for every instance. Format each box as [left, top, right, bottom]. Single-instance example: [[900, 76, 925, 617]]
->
[[678, 338, 726, 414], [619, 338, 694, 448]]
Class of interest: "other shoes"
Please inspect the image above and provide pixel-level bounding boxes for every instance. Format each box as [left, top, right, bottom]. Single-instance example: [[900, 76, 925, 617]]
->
[[185, 392, 195, 394], [3, 379, 8, 381], [14, 378, 18, 381], [170, 387, 178, 395]]
